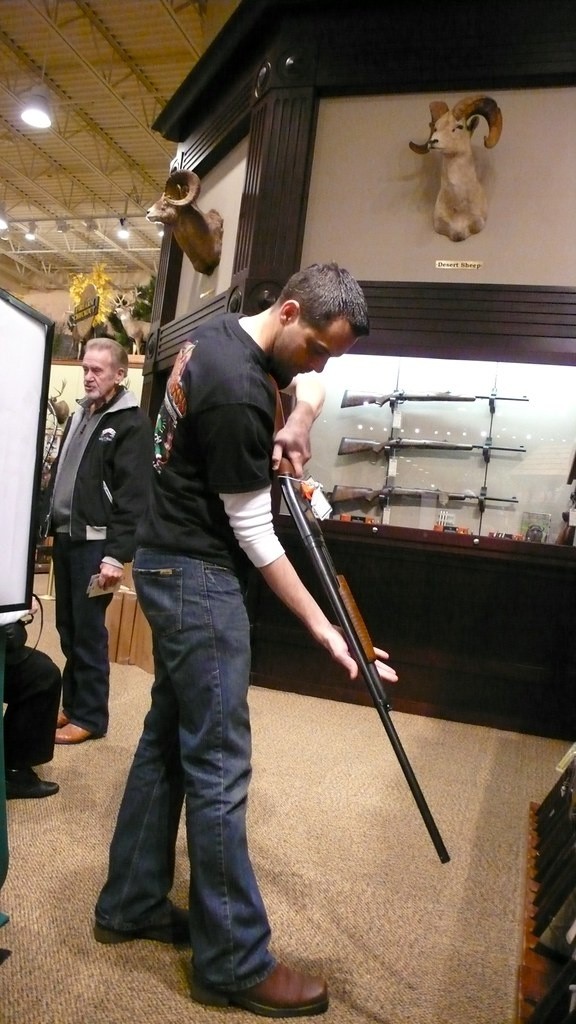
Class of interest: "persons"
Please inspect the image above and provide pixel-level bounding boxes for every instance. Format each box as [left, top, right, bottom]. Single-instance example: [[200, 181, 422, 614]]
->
[[39, 338, 152, 745], [93, 262, 398, 1019], [0, 597, 61, 798]]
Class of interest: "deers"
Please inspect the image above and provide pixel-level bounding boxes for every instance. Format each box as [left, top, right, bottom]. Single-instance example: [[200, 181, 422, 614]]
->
[[47, 379, 69, 424]]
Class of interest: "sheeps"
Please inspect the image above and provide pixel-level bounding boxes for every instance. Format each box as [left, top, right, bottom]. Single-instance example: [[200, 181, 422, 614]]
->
[[146, 169, 224, 276], [408, 95, 503, 242]]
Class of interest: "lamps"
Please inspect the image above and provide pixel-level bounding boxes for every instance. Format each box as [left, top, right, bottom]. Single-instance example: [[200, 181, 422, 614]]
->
[[20, 1, 52, 129], [25, 221, 37, 240], [56, 220, 68, 233], [84, 219, 99, 230]]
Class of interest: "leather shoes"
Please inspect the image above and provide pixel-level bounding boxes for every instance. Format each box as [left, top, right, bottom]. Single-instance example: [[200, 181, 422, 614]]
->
[[55, 723, 92, 744], [94, 906, 192, 945], [190, 962, 329, 1017], [5, 767, 59, 800], [56, 708, 70, 728]]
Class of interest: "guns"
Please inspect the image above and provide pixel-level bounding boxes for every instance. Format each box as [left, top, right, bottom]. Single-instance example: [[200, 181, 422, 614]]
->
[[269, 374, 452, 863], [337, 381, 529, 409], [338, 435, 528, 458], [330, 483, 522, 510]]
